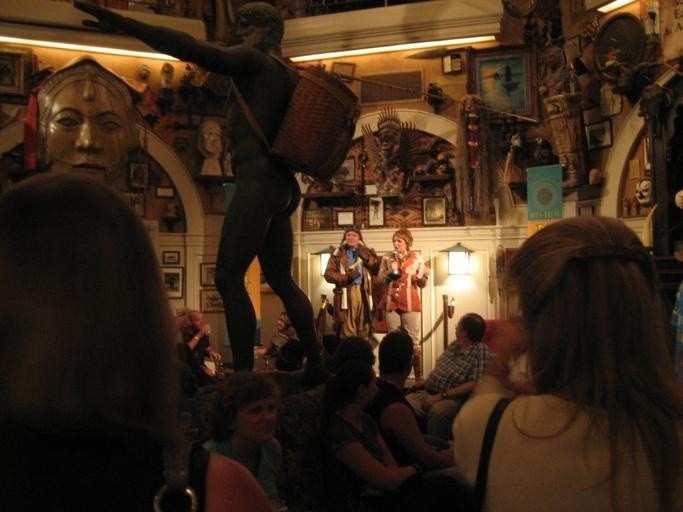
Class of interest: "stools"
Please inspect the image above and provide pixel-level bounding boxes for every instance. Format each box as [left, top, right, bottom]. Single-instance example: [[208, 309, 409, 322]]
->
[[256, 348, 270, 369]]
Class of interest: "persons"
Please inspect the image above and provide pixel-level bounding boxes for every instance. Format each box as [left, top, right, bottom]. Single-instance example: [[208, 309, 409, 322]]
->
[[33, 65, 143, 188], [74, 0, 322, 397], [1, 171, 683, 512]]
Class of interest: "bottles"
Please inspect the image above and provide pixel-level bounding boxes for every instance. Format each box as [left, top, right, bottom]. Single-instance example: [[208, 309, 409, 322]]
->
[[218, 362, 225, 379]]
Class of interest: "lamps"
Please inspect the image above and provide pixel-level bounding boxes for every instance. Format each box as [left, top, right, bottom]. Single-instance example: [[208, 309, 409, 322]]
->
[[583, 0, 637, 16], [438, 241, 472, 276], [310, 243, 338, 278]]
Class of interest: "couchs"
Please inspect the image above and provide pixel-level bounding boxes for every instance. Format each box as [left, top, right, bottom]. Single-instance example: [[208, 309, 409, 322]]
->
[[467, 317, 537, 393]]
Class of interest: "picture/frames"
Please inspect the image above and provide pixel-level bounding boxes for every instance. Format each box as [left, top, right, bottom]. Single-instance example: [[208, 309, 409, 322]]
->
[[199, 288, 225, 313], [584, 117, 616, 153], [465, 42, 542, 124], [336, 210, 356, 227], [199, 262, 217, 287], [260, 270, 274, 293], [505, 179, 529, 209], [578, 205, 596, 219], [0, 44, 33, 106], [561, 34, 585, 61], [362, 183, 378, 197], [329, 158, 356, 184], [367, 196, 386, 228], [332, 62, 357, 83], [420, 195, 448, 227], [117, 156, 186, 300]]
